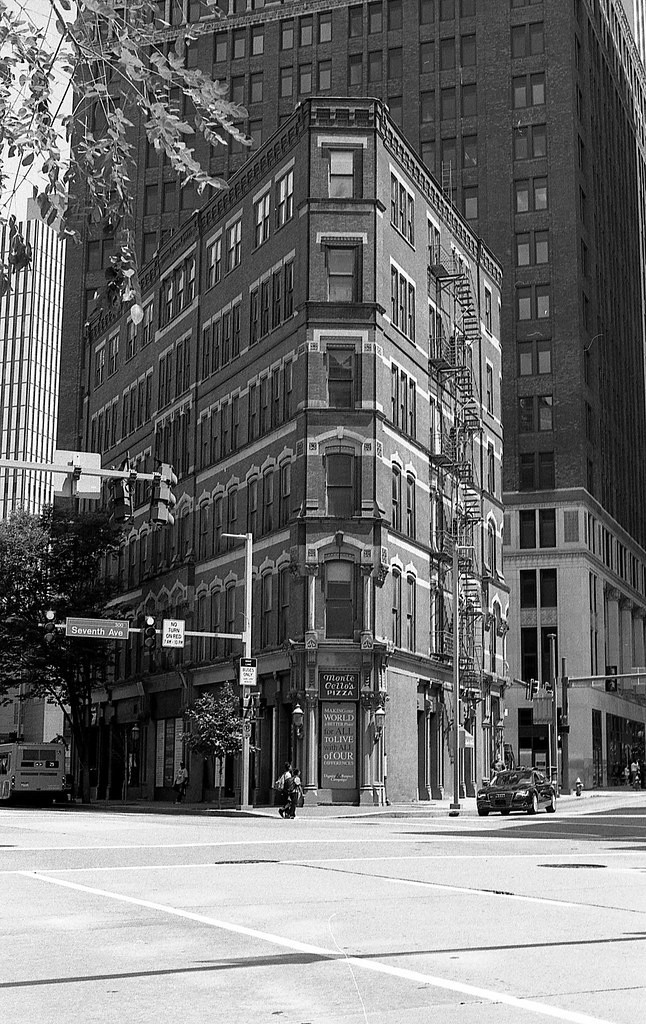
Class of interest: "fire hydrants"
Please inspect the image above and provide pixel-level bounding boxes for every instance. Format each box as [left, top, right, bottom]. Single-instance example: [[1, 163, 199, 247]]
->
[[575, 778, 584, 797]]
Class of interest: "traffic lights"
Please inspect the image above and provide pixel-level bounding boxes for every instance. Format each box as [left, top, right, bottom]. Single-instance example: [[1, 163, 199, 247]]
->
[[144, 615, 158, 651], [527, 678, 538, 702], [43, 610, 57, 647], [105, 469, 133, 523], [544, 683, 552, 692], [151, 462, 177, 529]]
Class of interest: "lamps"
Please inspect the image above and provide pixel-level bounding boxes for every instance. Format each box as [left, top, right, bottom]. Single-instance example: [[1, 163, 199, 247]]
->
[[291, 703, 306, 740], [373, 704, 386, 740], [131, 723, 139, 741]]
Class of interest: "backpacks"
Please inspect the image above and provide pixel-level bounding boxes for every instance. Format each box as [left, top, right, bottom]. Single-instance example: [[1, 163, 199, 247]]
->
[[284, 776, 300, 792], [275, 774, 286, 791]]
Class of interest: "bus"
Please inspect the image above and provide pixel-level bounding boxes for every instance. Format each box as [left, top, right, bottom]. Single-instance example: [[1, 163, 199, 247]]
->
[[0, 743, 67, 807]]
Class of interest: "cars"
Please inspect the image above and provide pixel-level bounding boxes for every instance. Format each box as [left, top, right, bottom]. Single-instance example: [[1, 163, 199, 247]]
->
[[476, 767, 558, 815]]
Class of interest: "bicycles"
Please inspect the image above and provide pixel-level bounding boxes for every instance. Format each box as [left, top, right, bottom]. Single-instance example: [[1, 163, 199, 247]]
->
[[635, 768, 641, 791]]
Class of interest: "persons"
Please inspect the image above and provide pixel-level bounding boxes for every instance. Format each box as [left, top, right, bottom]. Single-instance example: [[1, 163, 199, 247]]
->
[[630, 759, 645, 787], [172, 761, 188, 804], [490, 753, 506, 779], [612, 760, 621, 785], [622, 764, 630, 777], [278, 769, 303, 819], [283, 762, 293, 818]]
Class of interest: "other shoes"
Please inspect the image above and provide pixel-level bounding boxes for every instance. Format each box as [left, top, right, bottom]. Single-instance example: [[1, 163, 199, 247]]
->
[[279, 809, 284, 817], [290, 815, 295, 819]]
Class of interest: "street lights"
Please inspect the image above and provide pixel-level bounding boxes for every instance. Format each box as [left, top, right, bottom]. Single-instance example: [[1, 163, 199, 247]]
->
[[451, 544, 474, 809], [130, 722, 141, 802]]
